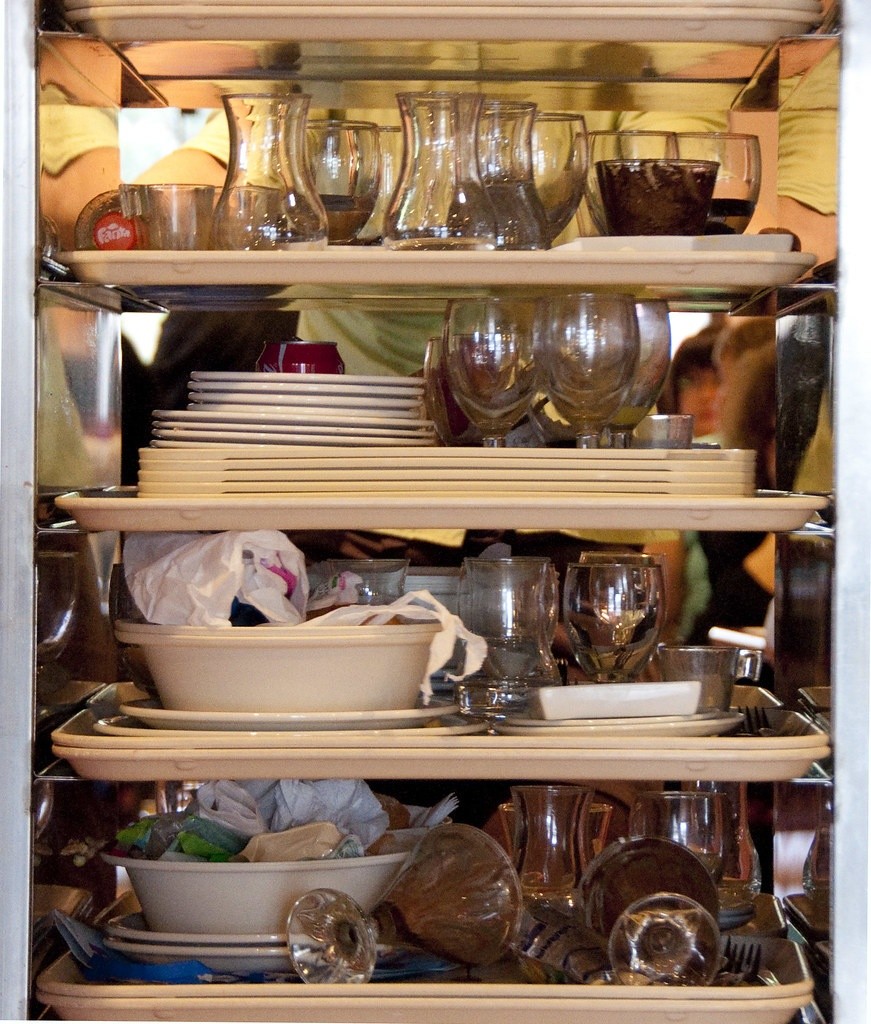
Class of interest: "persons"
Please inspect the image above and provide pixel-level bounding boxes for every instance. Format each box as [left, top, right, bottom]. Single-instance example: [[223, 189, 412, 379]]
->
[[122, 306, 516, 831], [298, 43, 832, 896]]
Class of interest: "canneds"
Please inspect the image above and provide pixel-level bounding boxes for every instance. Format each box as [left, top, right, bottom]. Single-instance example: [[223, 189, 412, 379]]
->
[[255, 329, 345, 374]]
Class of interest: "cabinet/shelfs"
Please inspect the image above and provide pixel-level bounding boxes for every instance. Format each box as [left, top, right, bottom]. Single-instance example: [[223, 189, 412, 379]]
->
[[32, 29, 841, 1021]]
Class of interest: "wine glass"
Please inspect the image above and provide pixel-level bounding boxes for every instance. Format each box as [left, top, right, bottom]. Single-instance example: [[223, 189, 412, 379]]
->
[[285, 822, 525, 984], [422, 290, 672, 449]]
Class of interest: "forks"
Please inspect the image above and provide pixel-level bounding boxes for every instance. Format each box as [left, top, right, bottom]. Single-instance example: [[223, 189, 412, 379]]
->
[[738, 705, 771, 737]]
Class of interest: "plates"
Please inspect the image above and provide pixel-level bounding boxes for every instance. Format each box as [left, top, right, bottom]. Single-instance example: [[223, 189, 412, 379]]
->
[[94, 699, 488, 738], [497, 715, 745, 736], [105, 911, 393, 975], [151, 371, 437, 449]]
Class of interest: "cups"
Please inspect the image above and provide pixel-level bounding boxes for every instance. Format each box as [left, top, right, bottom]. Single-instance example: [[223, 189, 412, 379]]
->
[[322, 549, 666, 715], [657, 646, 740, 713], [499, 784, 763, 988], [117, 87, 763, 253], [628, 412, 695, 449]]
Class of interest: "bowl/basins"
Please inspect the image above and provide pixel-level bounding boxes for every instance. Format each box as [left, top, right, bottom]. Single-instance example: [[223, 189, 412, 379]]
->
[[112, 616, 445, 713], [99, 804, 453, 934]]
